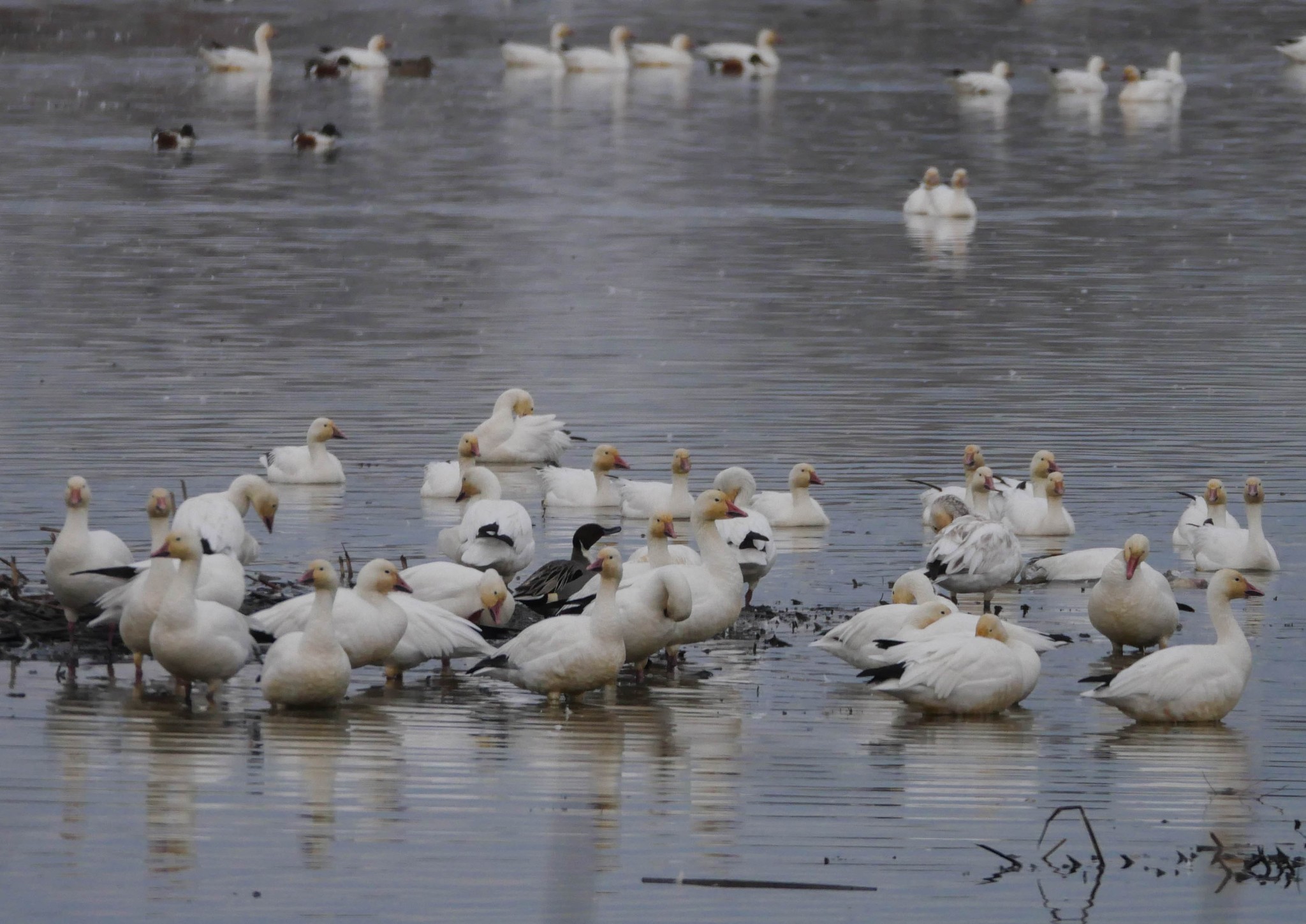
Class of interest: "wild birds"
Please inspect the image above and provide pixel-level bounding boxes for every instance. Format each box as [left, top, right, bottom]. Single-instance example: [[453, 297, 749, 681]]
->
[[903, 166, 978, 218], [200, 22, 279, 73], [946, 60, 1016, 95], [151, 123, 196, 150], [807, 445, 1124, 717], [1118, 51, 1188, 101], [1088, 534, 1196, 655], [291, 122, 340, 147], [1172, 479, 1241, 546], [1049, 55, 1111, 95], [1273, 35, 1306, 62], [45, 432, 831, 717], [499, 22, 785, 80], [473, 388, 588, 464], [258, 416, 349, 483], [1077, 568, 1266, 723], [1185, 476, 1281, 572], [305, 34, 436, 80]]
